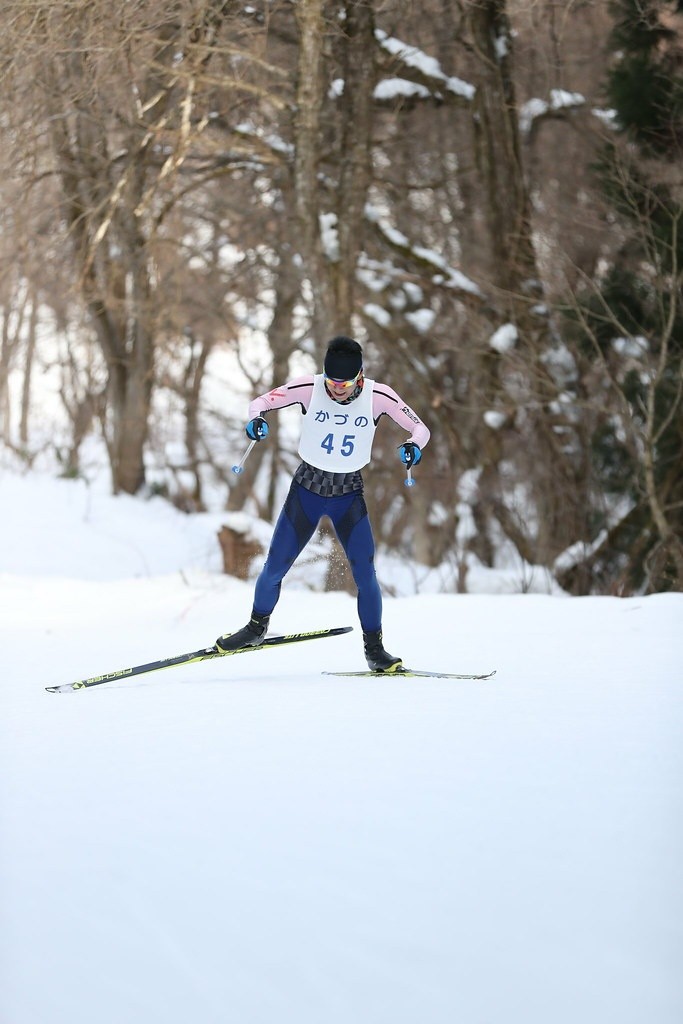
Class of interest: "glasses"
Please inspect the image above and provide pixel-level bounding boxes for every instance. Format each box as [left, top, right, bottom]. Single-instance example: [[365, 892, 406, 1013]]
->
[[323, 369, 361, 388]]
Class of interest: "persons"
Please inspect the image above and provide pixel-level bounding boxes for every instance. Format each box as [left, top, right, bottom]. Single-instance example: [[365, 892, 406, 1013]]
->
[[214, 335, 432, 674]]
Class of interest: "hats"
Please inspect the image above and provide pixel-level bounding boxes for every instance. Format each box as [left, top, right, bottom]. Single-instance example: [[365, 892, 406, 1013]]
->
[[324, 337, 362, 379]]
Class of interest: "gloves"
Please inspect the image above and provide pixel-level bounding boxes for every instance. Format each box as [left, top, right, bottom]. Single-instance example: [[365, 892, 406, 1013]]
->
[[397, 442, 421, 470], [245, 416, 269, 441]]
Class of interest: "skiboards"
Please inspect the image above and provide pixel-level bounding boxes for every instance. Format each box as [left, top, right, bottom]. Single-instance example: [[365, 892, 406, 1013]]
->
[[44, 625, 498, 694]]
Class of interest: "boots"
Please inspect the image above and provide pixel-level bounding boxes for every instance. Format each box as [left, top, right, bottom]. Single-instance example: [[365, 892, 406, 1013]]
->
[[215, 610, 270, 653], [363, 627, 402, 674]]
